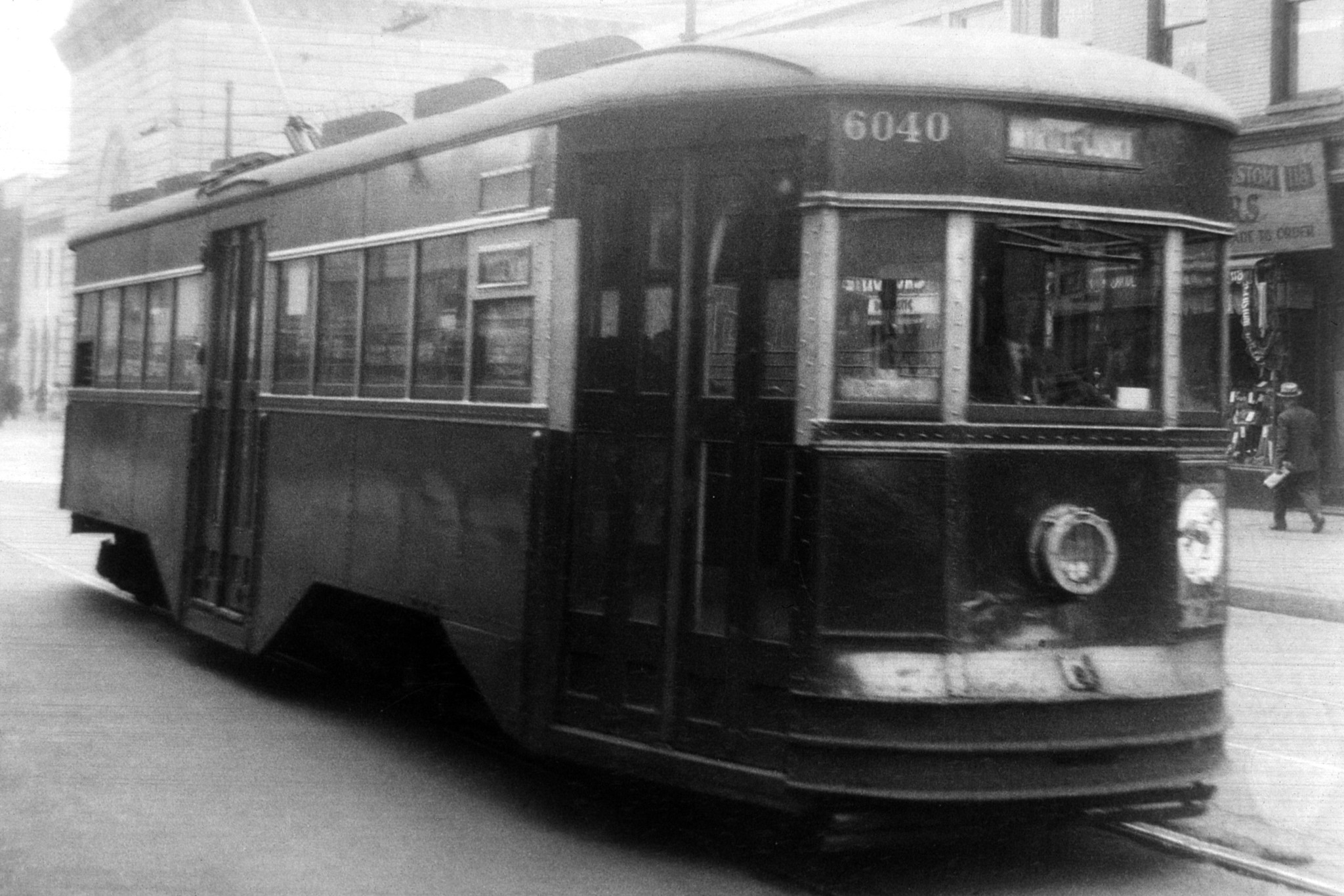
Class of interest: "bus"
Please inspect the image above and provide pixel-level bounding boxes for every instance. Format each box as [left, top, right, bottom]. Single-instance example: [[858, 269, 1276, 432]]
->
[[56, 36, 1245, 824]]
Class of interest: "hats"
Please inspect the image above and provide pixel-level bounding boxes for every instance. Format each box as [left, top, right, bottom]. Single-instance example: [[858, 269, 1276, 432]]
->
[[1275, 382, 1303, 398]]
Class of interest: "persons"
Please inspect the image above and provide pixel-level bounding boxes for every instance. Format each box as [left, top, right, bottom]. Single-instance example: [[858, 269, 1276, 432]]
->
[[1269, 382, 1326, 534], [1228, 380, 1269, 460], [972, 286, 1119, 409]]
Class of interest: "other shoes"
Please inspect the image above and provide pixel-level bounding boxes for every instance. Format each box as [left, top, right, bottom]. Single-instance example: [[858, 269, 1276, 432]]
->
[[1269, 521, 1286, 530], [1312, 514, 1324, 534]]
